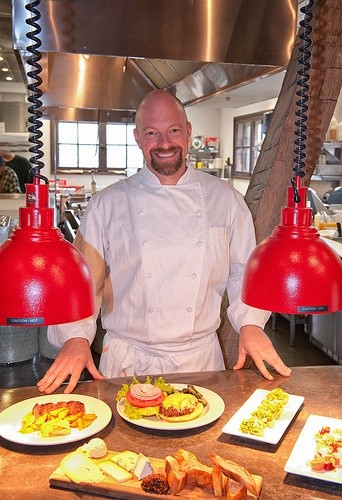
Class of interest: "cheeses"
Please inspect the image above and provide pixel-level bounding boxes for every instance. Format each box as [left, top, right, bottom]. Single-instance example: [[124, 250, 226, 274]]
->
[[61, 448, 154, 484]]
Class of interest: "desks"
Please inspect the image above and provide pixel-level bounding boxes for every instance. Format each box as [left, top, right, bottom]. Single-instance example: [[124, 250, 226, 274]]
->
[[0, 365, 342, 500]]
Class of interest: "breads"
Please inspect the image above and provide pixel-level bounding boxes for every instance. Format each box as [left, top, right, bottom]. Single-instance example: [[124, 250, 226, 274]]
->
[[165, 448, 259, 500]]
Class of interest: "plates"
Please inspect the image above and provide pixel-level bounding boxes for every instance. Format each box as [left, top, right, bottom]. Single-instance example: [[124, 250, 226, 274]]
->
[[284, 415, 342, 484], [49, 446, 263, 500], [116, 383, 226, 431], [0, 393, 112, 446], [221, 388, 305, 444]]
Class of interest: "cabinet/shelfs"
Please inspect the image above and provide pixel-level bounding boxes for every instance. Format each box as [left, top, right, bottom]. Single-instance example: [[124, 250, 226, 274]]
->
[[308, 139, 342, 181]]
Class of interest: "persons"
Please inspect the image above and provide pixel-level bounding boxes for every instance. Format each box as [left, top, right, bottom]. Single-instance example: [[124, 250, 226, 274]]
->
[[0, 152, 33, 194], [37, 89, 292, 395]]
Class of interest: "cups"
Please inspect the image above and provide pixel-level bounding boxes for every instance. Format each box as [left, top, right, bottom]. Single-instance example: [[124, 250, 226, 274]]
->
[[215, 157, 222, 169], [196, 162, 202, 169]]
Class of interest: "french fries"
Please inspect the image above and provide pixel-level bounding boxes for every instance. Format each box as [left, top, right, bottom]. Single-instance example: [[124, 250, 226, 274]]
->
[[19, 407, 97, 435]]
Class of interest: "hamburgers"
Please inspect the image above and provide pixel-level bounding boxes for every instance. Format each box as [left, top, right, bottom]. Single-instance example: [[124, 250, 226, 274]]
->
[[117, 375, 204, 422]]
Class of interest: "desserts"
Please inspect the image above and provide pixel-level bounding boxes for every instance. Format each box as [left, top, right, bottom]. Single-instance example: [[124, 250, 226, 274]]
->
[[309, 425, 342, 472], [240, 388, 289, 436]]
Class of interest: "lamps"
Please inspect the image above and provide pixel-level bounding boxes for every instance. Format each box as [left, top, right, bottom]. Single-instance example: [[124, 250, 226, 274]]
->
[[0, 0, 96, 326], [242, 0, 342, 315]]
